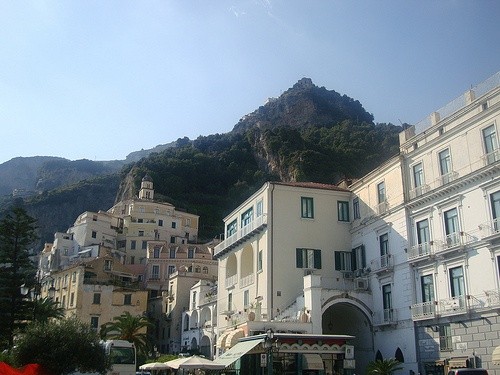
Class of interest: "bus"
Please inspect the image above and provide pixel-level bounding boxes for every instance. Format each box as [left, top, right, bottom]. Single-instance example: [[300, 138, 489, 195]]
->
[[90, 339, 137, 375]]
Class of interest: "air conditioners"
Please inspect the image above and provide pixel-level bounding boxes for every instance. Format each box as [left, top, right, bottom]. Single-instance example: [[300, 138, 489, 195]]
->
[[343, 272, 353, 279], [355, 279, 368, 290], [304, 269, 315, 276]]
